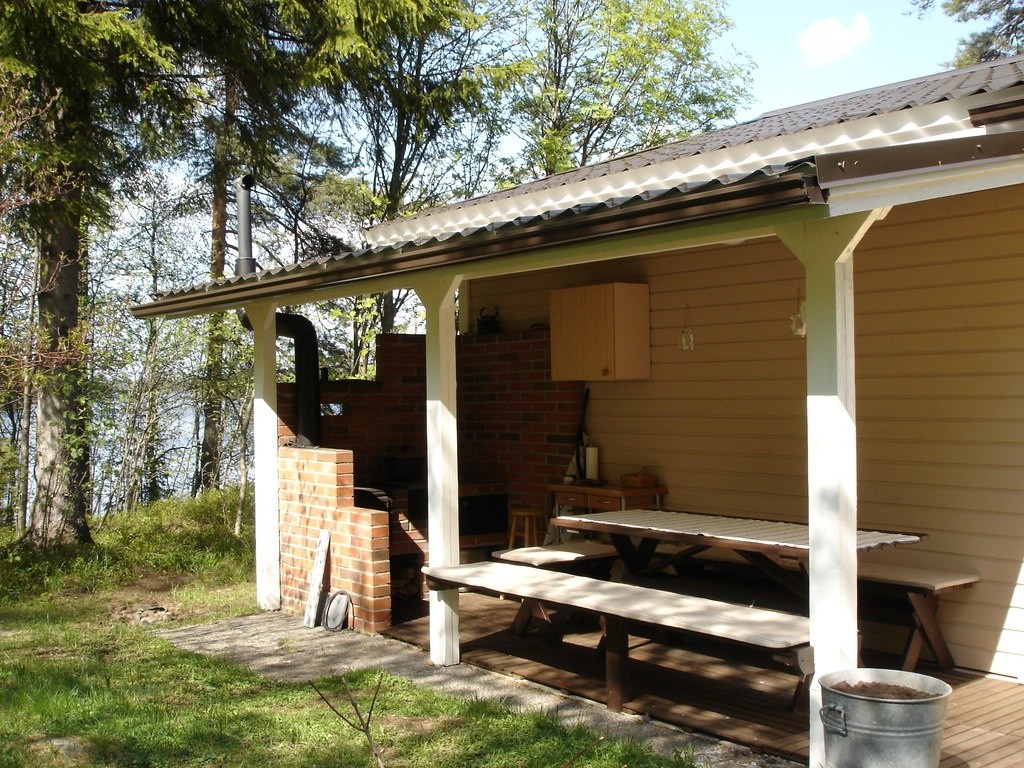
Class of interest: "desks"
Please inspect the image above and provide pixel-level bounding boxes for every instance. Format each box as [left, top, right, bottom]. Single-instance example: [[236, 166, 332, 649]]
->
[[551, 507, 927, 714], [546, 483, 667, 545]]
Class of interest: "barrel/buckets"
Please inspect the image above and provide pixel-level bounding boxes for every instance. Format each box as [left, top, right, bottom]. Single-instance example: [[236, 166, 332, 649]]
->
[[818, 666, 954, 768]]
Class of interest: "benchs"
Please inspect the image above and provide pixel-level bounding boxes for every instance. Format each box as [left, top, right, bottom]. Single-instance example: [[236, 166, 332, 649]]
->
[[422, 561, 813, 712], [772, 557, 981, 677], [491, 538, 620, 637]]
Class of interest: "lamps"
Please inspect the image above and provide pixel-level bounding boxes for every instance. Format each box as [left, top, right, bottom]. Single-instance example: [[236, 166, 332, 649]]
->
[[789, 276, 807, 337], [680, 305, 696, 351]]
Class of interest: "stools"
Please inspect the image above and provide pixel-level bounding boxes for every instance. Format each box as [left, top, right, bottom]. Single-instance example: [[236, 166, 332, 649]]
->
[[507, 509, 544, 550]]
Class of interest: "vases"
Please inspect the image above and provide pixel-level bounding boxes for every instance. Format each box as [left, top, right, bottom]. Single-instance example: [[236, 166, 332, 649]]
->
[[818, 668, 954, 767]]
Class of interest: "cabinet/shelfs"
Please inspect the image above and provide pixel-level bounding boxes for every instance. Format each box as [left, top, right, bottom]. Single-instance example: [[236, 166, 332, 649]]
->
[[548, 282, 651, 383]]
